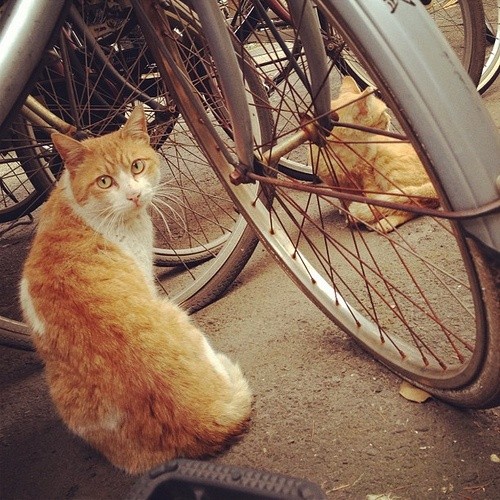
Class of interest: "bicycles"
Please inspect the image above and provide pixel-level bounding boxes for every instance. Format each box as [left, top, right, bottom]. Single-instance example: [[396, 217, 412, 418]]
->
[[0, 1, 500, 416]]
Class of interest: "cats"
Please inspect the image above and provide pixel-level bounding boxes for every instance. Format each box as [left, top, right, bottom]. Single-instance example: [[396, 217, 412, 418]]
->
[[16, 103, 255, 478], [309, 75, 443, 233]]
